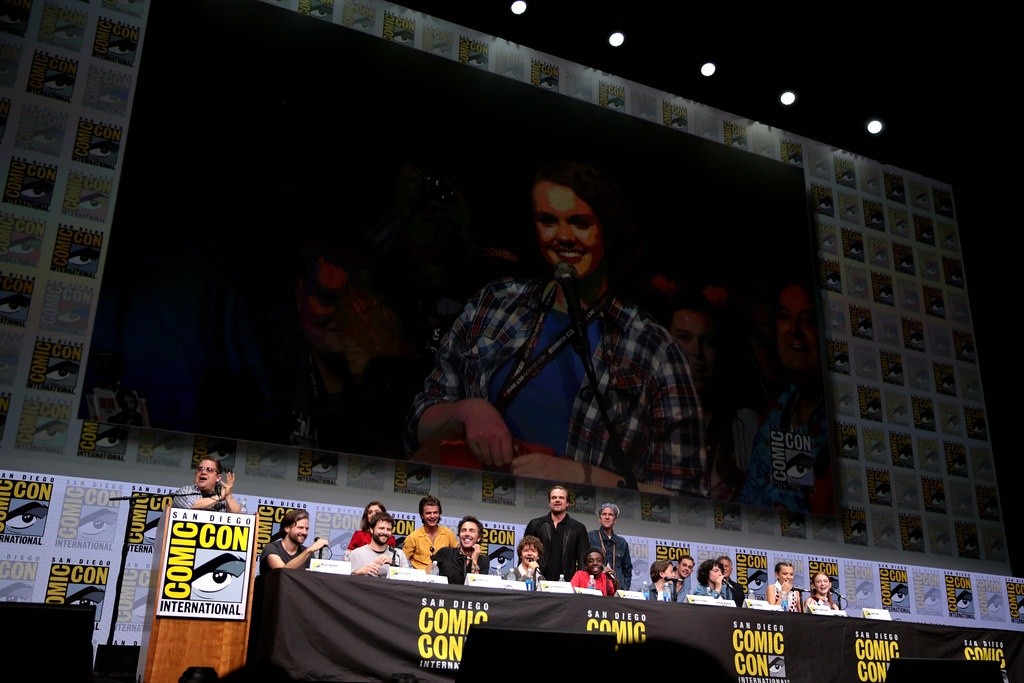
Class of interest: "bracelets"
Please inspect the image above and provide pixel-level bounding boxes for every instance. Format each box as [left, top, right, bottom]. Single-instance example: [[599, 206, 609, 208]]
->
[[781, 591, 787, 595], [471, 566, 480, 570]]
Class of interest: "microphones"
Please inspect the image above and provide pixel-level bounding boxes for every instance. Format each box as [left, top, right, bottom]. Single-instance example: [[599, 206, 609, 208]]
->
[[790, 587, 804, 592], [829, 588, 846, 599], [315, 537, 328, 547], [215, 483, 221, 496], [664, 577, 684, 582], [529, 558, 541, 574], [552, 262, 592, 354], [601, 564, 616, 579], [722, 578, 736, 592], [388, 546, 400, 557], [458, 552, 471, 561]]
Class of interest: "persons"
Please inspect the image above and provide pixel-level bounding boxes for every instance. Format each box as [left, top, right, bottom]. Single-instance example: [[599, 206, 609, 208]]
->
[[279, 152, 835, 514], [647, 555, 695, 603], [504, 535, 546, 581], [694, 556, 745, 607], [587, 502, 633, 590], [766, 562, 802, 612], [345, 496, 490, 586], [803, 572, 839, 613], [570, 549, 619, 597], [173, 456, 241, 513], [259, 509, 329, 572], [523, 486, 592, 581]]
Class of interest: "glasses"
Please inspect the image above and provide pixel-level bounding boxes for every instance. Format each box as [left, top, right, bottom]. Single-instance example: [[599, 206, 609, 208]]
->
[[430, 547, 436, 561], [198, 467, 219, 474], [312, 284, 344, 307]]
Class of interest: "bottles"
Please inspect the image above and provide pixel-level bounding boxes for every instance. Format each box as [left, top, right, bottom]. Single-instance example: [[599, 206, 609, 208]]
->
[[524, 568, 534, 591], [641, 581, 650, 600], [558, 574, 566, 582], [705, 587, 712, 596], [780, 591, 789, 611], [662, 583, 671, 602], [430, 561, 439, 576], [747, 590, 755, 600], [507, 568, 516, 581], [493, 566, 502, 579], [587, 575, 596, 590]]
